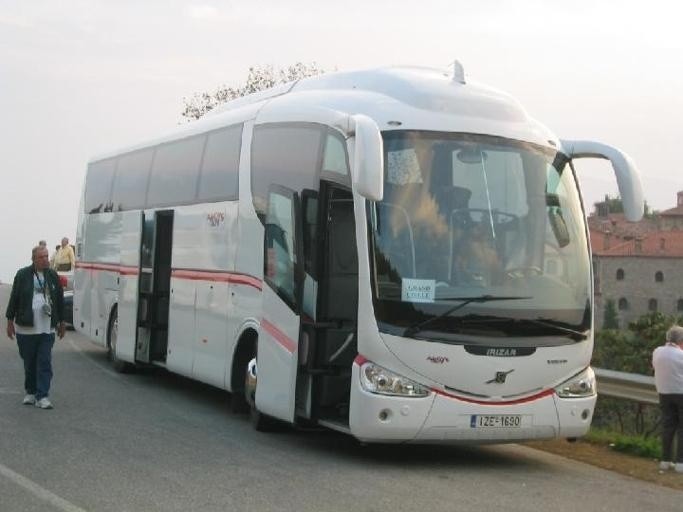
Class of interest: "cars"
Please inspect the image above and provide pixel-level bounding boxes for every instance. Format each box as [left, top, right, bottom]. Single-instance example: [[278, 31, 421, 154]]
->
[[55, 269, 72, 322]]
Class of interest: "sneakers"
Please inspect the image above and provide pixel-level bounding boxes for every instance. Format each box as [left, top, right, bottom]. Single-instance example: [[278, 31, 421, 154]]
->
[[22, 392, 37, 405], [659, 461, 683, 471], [34, 396, 54, 409]]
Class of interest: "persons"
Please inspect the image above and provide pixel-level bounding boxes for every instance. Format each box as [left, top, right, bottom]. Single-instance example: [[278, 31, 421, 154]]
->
[[38, 237, 74, 271], [3, 244, 66, 410], [651, 324, 682, 475]]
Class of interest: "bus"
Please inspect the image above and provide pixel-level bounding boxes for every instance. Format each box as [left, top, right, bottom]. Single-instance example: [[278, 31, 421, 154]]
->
[[69, 56, 650, 459]]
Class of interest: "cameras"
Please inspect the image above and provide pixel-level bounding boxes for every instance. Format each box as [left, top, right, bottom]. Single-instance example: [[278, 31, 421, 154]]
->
[[43, 304, 52, 316]]
